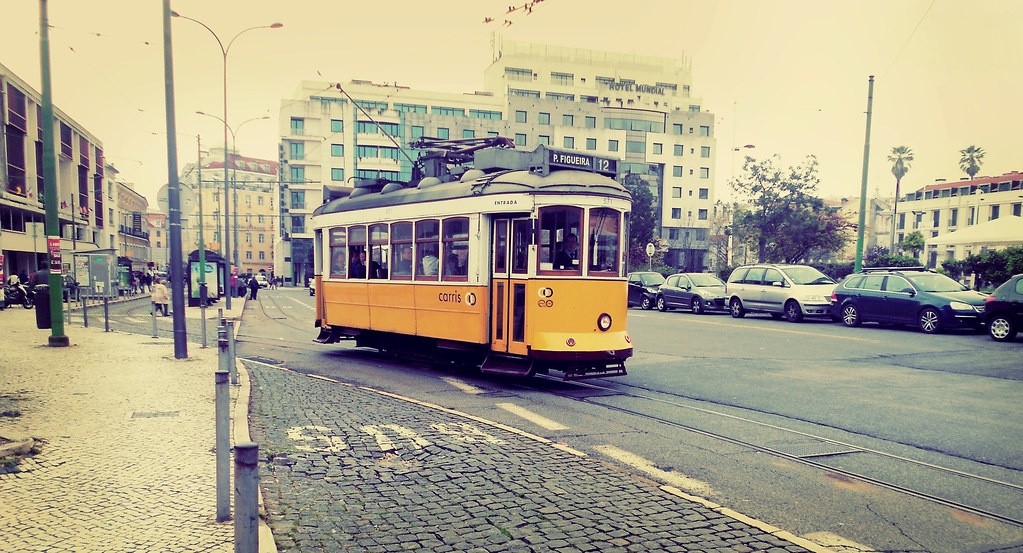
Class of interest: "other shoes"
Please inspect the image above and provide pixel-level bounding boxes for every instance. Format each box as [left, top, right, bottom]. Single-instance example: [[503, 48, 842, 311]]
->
[[150, 312, 152, 316], [164, 313, 171, 316]]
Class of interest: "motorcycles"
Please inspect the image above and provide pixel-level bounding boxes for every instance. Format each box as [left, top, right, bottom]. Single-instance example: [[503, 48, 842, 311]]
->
[[4, 280, 34, 309]]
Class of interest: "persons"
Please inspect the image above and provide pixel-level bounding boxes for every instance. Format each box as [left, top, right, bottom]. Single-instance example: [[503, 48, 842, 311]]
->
[[422, 245, 439, 275], [229, 267, 248, 297], [18, 269, 29, 284], [351, 249, 383, 279], [131, 272, 138, 294], [153, 273, 159, 284], [334, 250, 345, 271], [396, 246, 412, 274], [446, 253, 464, 275], [977, 272, 982, 291], [518, 251, 527, 268], [7, 270, 21, 286], [146, 273, 151, 292], [248, 277, 260, 300], [268, 275, 284, 290], [309, 275, 315, 296], [150, 277, 171, 316], [30, 261, 49, 288], [65, 270, 80, 295], [139, 272, 146, 293], [554, 233, 580, 270]]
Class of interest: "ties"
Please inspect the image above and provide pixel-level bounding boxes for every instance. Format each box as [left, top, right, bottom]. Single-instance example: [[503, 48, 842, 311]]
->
[[570, 253, 574, 259]]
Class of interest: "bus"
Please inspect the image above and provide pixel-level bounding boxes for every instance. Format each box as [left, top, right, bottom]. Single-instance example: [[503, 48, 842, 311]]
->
[[313, 83, 635, 381]]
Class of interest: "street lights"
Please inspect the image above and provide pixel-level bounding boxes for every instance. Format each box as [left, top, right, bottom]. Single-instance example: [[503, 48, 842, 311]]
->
[[171, 11, 284, 312], [195, 111, 270, 299]]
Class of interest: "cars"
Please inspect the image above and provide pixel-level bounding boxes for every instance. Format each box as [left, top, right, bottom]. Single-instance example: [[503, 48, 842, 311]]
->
[[308, 279, 315, 297], [255, 276, 267, 288], [655, 273, 727, 315], [61, 274, 80, 303], [984, 274, 1023, 343], [626, 272, 666, 310]]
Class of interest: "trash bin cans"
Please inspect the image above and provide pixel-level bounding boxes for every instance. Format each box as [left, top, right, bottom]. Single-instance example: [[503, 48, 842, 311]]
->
[[35, 284, 51, 329]]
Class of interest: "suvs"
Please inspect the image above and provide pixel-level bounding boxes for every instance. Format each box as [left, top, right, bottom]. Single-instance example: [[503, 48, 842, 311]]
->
[[831, 267, 991, 334], [724, 263, 839, 323]]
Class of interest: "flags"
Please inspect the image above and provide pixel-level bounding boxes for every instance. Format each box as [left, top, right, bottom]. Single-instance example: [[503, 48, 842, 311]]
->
[[78, 206, 93, 215]]
